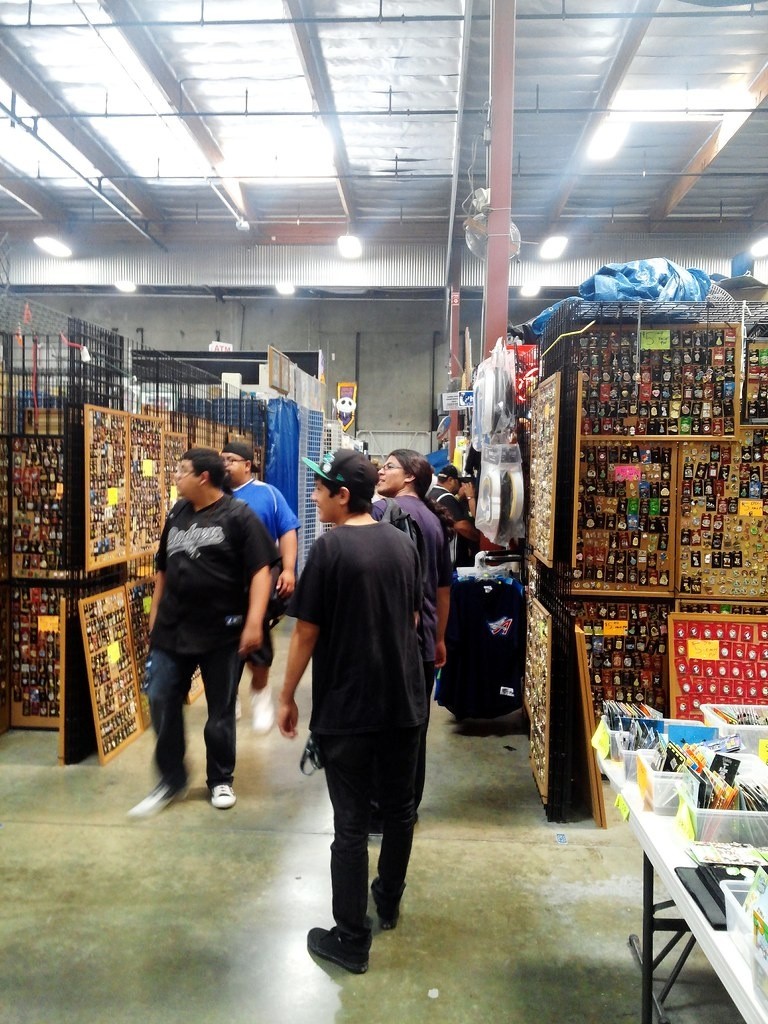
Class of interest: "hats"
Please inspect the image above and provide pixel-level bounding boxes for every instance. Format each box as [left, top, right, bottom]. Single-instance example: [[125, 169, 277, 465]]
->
[[301, 449, 378, 498], [222, 442, 260, 473], [438, 466, 468, 482]]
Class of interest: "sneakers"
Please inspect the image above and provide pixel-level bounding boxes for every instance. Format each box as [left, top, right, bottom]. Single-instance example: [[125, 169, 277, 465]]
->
[[210, 785, 237, 809], [308, 928, 369, 973], [371, 876, 398, 930], [235, 694, 242, 720], [251, 682, 274, 732]]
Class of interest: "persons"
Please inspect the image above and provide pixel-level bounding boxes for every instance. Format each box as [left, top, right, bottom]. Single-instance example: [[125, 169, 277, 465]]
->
[[132, 442, 302, 816], [367, 461, 485, 546], [367, 450, 453, 836], [279, 449, 428, 974]]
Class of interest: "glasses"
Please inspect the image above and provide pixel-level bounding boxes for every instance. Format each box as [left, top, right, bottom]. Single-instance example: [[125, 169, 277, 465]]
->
[[381, 464, 407, 470], [224, 459, 247, 465], [176, 469, 196, 478]]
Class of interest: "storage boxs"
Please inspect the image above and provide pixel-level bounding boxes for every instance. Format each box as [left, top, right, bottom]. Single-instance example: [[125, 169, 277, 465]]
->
[[600, 704, 768, 1014]]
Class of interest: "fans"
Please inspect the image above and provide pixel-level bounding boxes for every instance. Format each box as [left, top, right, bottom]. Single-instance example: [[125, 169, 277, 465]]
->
[[698, 285, 738, 322], [465, 210, 521, 262]]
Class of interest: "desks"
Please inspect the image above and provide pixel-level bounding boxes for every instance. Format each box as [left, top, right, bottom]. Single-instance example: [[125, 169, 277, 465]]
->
[[600, 758, 768, 1024]]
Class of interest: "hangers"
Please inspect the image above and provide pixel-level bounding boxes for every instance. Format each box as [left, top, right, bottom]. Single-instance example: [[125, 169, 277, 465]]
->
[[454, 554, 510, 586]]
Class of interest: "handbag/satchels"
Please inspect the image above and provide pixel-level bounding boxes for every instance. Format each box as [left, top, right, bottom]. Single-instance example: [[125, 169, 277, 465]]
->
[[378, 498, 428, 584]]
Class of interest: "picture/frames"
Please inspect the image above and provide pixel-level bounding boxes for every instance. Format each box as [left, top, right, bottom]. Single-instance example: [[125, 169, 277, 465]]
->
[[268, 346, 290, 394]]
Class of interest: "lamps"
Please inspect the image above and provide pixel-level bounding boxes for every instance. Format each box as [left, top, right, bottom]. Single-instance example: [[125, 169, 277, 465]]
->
[[590, 117, 631, 161], [34, 234, 72, 257], [338, 236, 363, 257], [539, 236, 568, 260]]
